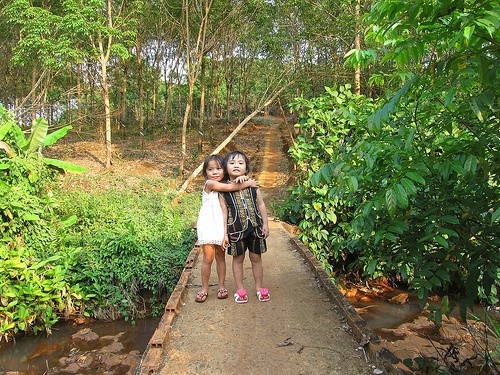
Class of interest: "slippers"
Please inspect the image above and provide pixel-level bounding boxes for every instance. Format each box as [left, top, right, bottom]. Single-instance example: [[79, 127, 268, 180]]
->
[[233, 289, 248, 303], [217, 288, 228, 299], [256, 288, 270, 302], [195, 292, 208, 302]]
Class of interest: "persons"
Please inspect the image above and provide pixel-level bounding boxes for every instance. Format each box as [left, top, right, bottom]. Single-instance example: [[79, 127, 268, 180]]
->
[[194, 154, 258, 303], [218, 151, 272, 303]]
